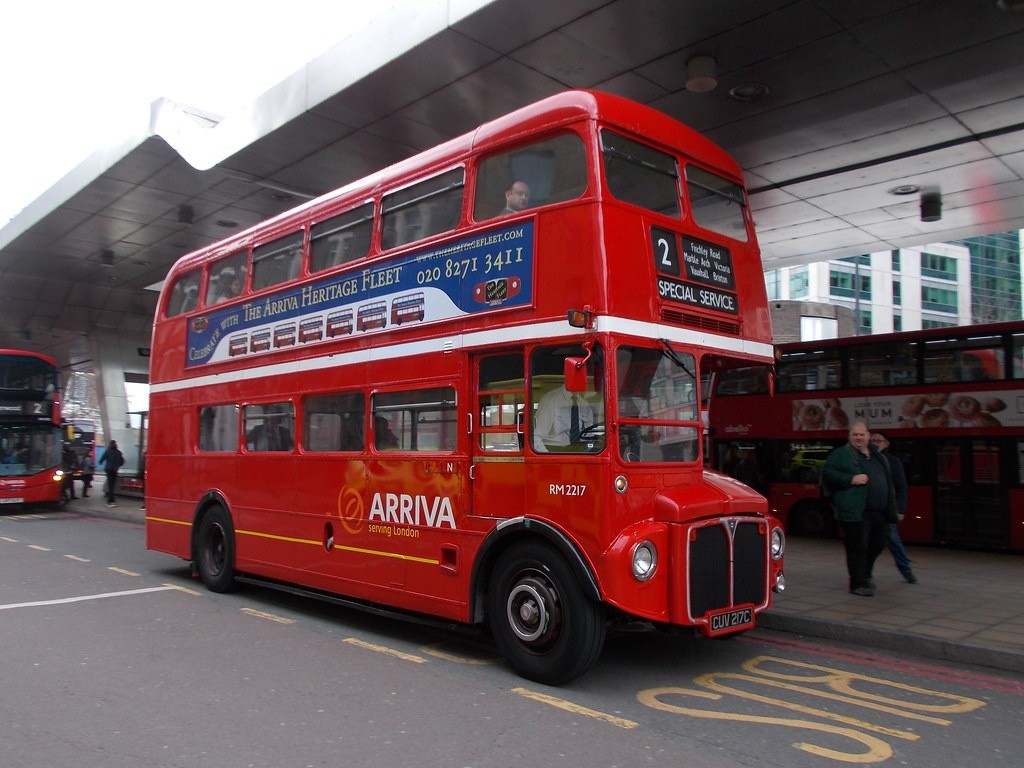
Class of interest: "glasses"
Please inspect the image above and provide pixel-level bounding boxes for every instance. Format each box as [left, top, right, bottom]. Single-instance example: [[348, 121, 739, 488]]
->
[[871, 438, 885, 445]]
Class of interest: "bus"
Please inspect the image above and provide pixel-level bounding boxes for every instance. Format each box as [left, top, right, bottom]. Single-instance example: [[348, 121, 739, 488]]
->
[[131, 85, 789, 691], [0, 348, 75, 514], [61, 418, 96, 480], [708, 317, 1023, 551]]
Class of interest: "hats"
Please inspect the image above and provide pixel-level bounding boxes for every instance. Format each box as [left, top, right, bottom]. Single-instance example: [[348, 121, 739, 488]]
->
[[267, 406, 286, 416]]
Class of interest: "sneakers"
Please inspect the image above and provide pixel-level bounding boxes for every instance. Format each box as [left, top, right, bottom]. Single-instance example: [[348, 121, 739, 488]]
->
[[905, 573, 917, 583], [864, 579, 877, 589], [850, 585, 873, 597]]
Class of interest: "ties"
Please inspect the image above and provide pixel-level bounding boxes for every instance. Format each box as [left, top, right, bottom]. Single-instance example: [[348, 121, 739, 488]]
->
[[271, 429, 281, 451], [568, 394, 581, 450]]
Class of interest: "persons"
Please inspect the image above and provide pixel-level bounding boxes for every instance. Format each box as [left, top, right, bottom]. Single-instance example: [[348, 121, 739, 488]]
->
[[0, 431, 56, 474], [62, 441, 80, 500], [494, 178, 530, 216], [99, 439, 125, 508], [215, 275, 244, 303], [81, 449, 97, 498], [244, 406, 292, 451], [750, 347, 997, 394], [821, 422, 898, 596], [132, 448, 146, 511], [373, 417, 402, 451], [865, 431, 919, 587], [533, 383, 595, 454]]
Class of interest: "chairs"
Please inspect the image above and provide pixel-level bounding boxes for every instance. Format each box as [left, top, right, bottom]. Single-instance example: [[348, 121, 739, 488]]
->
[[516, 405, 599, 452]]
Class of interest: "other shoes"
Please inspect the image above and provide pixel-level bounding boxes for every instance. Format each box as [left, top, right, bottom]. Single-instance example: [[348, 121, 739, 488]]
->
[[137, 504, 147, 511], [106, 502, 118, 509], [71, 496, 80, 501], [82, 494, 90, 498]]
